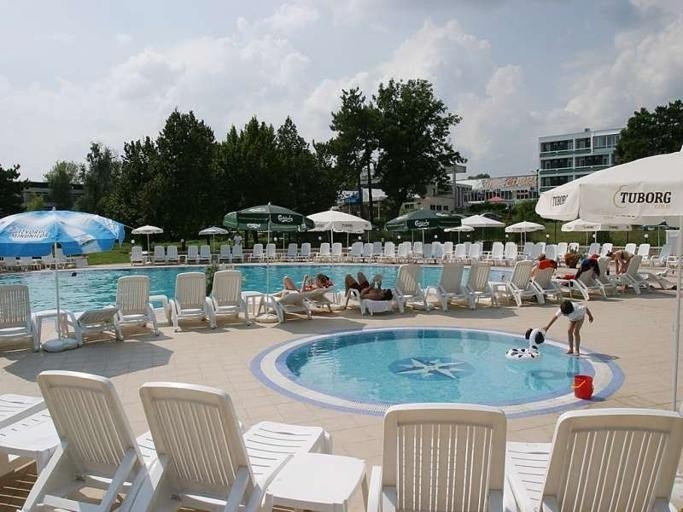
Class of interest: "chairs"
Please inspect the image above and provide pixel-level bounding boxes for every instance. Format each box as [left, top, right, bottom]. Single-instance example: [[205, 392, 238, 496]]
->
[[131, 382, 332, 512], [0, 393, 62, 480], [365, 403, 508, 512], [505, 408, 683, 512], [21, 370, 244, 512]]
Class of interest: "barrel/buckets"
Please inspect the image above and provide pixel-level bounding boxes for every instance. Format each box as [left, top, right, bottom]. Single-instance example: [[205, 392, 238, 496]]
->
[[573, 375, 593, 398]]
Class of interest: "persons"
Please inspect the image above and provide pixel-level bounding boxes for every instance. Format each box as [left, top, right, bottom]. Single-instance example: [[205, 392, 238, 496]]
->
[[605, 249, 636, 294], [530, 253, 556, 280], [556, 253, 600, 287], [271, 271, 394, 301], [545, 298, 593, 356]]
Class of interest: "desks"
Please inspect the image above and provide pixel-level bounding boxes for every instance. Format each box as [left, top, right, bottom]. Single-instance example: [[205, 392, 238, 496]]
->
[[264, 453, 370, 512]]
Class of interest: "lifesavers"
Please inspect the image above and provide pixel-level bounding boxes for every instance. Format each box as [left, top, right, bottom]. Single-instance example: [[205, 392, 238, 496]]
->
[[507, 329, 548, 362]]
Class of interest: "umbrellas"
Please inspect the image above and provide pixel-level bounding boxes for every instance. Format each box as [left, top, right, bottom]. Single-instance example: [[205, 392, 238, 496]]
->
[[535, 145, 683, 411], [561, 217, 632, 245], [1, 207, 130, 340]]
